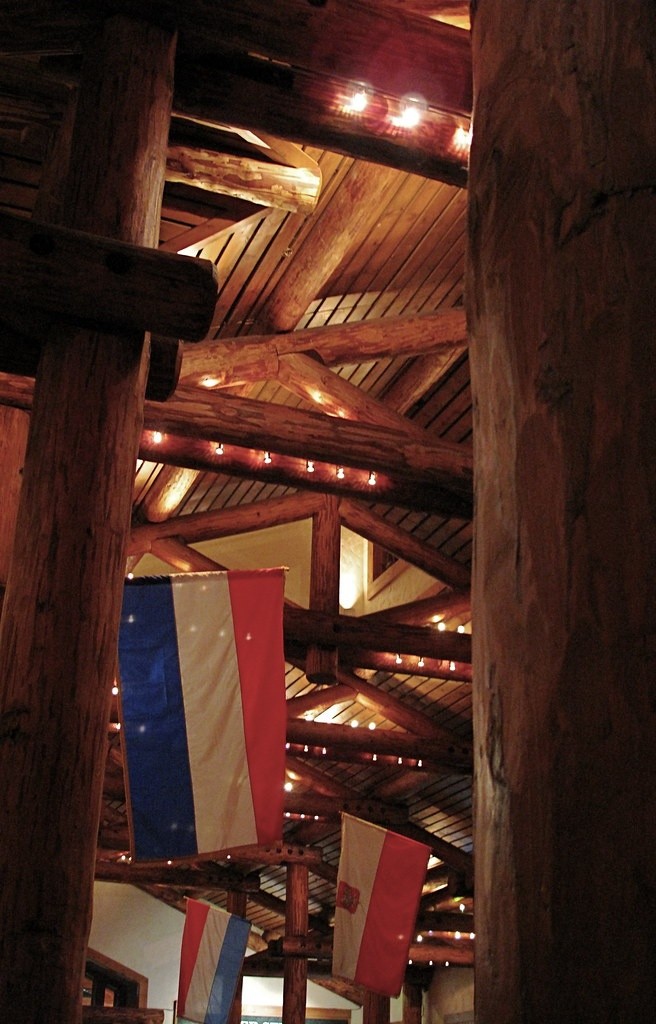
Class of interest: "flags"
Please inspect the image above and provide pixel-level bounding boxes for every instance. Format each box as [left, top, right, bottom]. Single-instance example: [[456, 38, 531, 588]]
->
[[116, 567, 288, 867], [176, 898, 252, 1024], [332, 811, 431, 999]]
